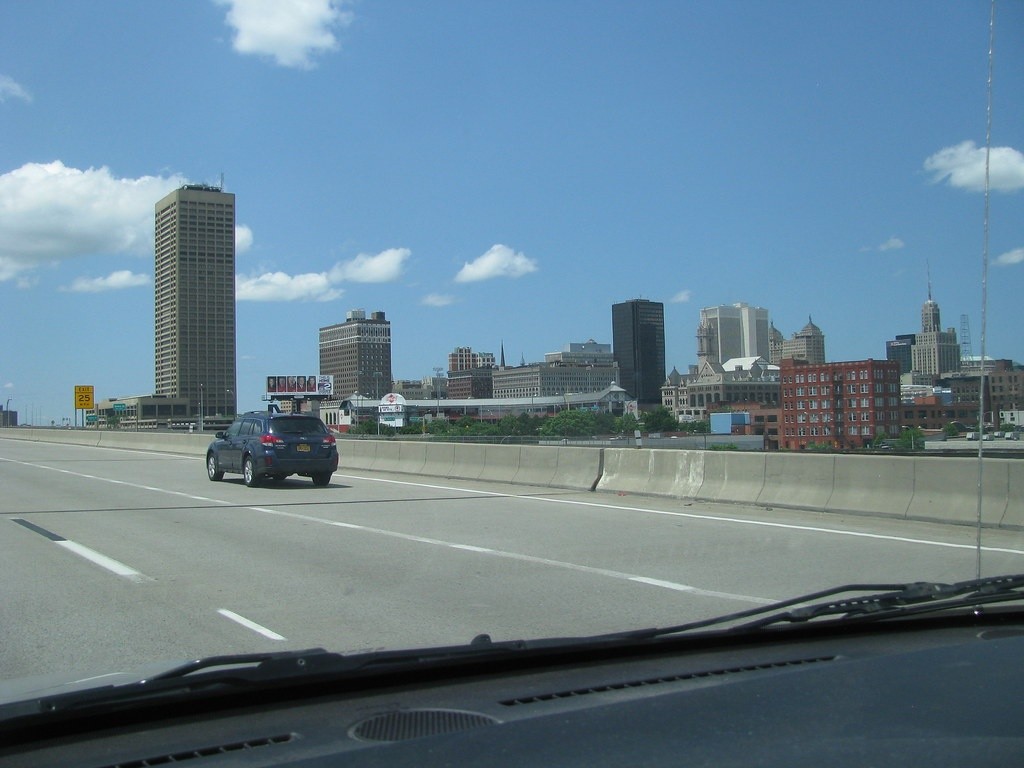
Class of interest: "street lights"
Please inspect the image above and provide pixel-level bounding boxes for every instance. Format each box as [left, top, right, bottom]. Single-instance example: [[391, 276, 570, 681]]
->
[[199, 402, 201, 431], [201, 384, 205, 431], [7, 398, 12, 428], [902, 426, 915, 450], [354, 391, 360, 428]]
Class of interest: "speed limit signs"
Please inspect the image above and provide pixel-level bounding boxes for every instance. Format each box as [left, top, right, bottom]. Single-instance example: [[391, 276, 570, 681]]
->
[[74, 385, 94, 410]]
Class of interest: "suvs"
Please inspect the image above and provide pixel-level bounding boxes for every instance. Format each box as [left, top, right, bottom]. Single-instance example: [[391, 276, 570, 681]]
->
[[206, 411, 339, 488]]
